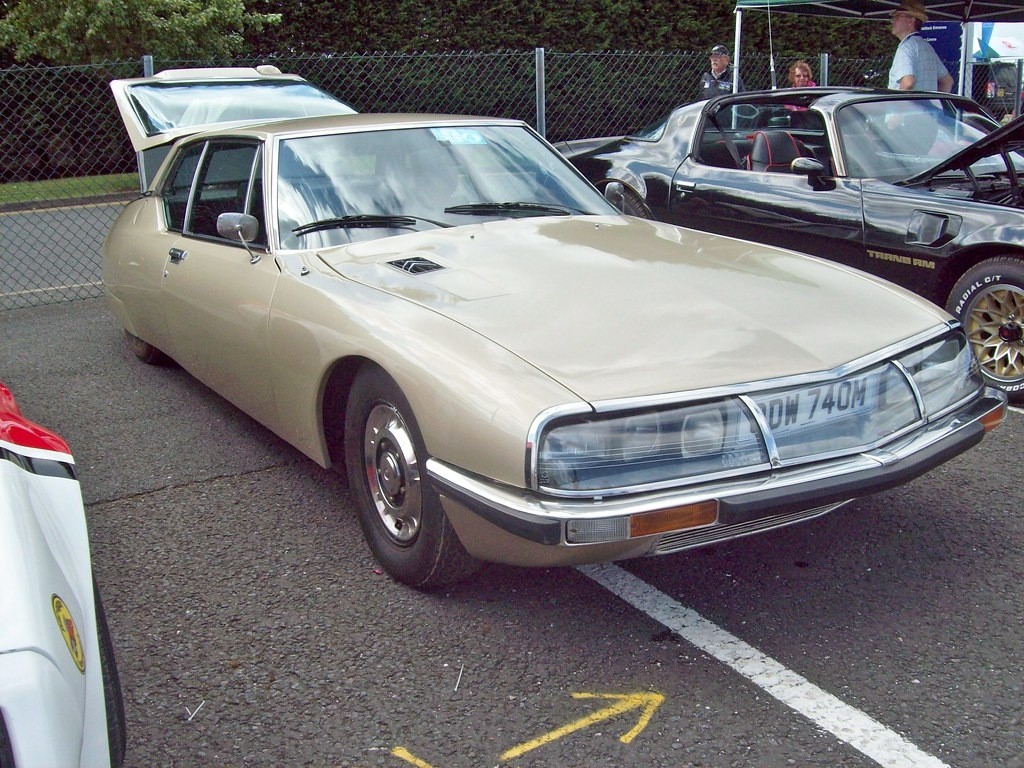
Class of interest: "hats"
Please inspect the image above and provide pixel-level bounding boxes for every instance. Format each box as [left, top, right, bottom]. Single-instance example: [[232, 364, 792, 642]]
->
[[706, 45, 729, 55], [889, 0, 928, 24]]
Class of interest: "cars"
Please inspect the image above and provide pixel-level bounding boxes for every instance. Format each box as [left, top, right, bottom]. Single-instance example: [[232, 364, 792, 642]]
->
[[100, 65, 1005, 591], [1, 388, 128, 768], [535, 85, 1024, 410]]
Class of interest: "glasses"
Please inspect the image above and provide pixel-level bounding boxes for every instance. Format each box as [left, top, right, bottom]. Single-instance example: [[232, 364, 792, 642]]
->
[[794, 74, 809, 78]]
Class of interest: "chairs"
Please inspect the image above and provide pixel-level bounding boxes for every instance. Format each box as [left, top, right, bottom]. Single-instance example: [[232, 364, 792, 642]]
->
[[235, 179, 266, 236], [742, 131, 801, 173], [826, 132, 886, 173], [374, 146, 459, 211]]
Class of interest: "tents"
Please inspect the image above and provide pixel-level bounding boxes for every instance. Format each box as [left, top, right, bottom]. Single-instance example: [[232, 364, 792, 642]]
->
[[731, 0, 1024, 143]]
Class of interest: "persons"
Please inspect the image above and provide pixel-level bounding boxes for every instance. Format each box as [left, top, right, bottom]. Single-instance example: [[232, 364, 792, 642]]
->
[[878, 0, 954, 154], [783, 61, 818, 111], [698, 45, 746, 131]]
